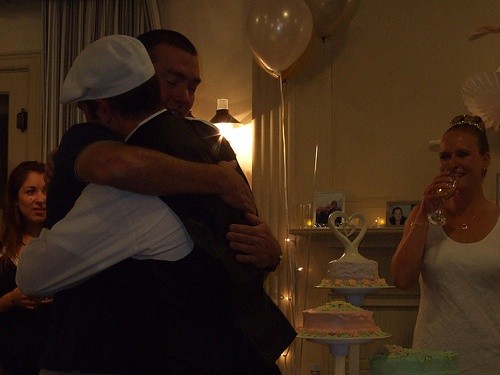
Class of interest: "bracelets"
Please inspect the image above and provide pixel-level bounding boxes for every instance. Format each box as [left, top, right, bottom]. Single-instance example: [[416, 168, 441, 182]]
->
[[264, 250, 283, 272], [410, 222, 430, 227]]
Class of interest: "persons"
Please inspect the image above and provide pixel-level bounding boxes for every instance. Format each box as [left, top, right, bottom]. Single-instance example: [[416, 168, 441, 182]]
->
[[316, 200, 342, 227], [389, 207, 407, 225], [16, 29, 297, 375], [0, 160, 54, 375], [390, 115, 500, 375]]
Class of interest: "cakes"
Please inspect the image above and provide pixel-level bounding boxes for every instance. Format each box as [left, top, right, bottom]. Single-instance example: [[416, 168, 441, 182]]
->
[[317, 210, 389, 288], [369, 344, 459, 375], [296, 301, 388, 339]]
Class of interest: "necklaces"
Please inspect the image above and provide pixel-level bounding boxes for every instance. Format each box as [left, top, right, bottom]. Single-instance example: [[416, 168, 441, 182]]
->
[[452, 200, 486, 230]]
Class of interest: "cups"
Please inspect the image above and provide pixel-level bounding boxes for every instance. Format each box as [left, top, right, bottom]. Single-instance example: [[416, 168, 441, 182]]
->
[[297, 204, 311, 228]]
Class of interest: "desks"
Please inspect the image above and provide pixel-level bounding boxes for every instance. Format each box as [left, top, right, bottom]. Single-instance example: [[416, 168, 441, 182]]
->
[[296, 334, 392, 375], [313, 285, 396, 375]]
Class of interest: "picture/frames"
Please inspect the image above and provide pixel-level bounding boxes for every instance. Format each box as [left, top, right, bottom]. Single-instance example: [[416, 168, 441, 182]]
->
[[312, 191, 345, 226], [384, 200, 420, 227]]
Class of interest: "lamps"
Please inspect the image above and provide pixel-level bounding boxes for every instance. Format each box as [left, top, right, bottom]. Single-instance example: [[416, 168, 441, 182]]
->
[[210, 98, 242, 125]]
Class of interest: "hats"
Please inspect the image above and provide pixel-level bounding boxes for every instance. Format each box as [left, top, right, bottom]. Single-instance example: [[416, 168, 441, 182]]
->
[[59, 35, 156, 105]]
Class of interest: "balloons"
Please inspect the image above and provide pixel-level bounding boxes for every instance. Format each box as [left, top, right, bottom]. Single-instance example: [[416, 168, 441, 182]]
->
[[246, 0, 360, 87]]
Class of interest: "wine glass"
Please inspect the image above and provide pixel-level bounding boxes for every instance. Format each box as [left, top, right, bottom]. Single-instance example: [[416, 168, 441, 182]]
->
[[427, 166, 459, 226]]
